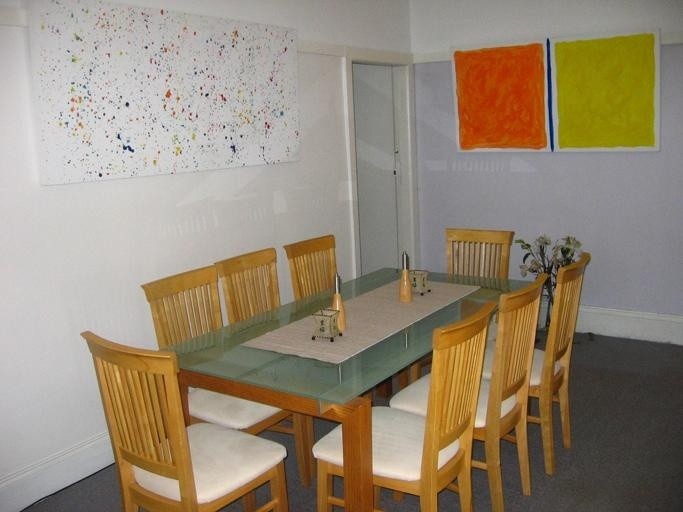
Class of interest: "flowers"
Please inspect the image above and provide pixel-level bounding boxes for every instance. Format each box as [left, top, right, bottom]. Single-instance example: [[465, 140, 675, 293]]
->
[[515, 235, 581, 304]]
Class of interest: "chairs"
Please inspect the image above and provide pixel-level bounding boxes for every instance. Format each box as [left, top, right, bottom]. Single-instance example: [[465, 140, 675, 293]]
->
[[216, 247, 283, 324], [481, 252, 590, 475], [312, 302, 499, 512], [140, 261, 310, 489], [389, 273, 548, 511], [284, 234, 340, 301], [81, 329, 288, 512], [444, 227, 515, 277]]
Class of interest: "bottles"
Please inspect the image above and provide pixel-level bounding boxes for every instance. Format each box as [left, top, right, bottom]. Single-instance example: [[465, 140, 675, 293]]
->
[[329, 273, 346, 335], [397, 251, 413, 305]]
